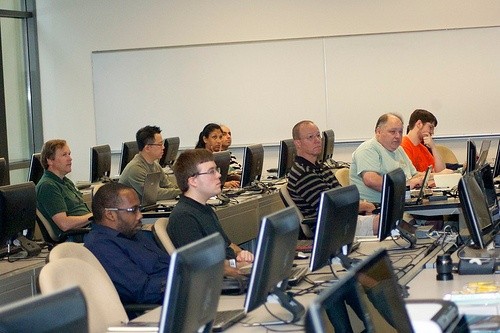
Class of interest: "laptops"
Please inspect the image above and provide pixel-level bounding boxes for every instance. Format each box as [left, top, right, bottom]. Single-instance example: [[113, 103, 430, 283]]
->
[[477, 139, 491, 167], [406, 166, 432, 206], [138, 171, 162, 210]]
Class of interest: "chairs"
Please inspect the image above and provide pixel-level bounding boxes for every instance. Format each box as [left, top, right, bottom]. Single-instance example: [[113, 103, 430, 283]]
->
[[151, 216, 177, 255], [48, 241, 130, 324], [279, 183, 317, 239], [39, 258, 124, 333]]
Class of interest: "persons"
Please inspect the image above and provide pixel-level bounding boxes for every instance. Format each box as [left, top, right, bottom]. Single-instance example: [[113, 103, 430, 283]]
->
[[349, 114, 452, 232], [33, 139, 94, 244], [287, 120, 380, 237], [194, 123, 240, 190], [218, 125, 243, 179], [166, 149, 255, 278], [83, 182, 171, 321], [117, 125, 184, 205], [398, 108, 446, 174]]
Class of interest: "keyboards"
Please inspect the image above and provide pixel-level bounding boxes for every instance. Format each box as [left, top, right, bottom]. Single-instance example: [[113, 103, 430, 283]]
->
[[214, 308, 244, 333], [352, 241, 360, 250], [288, 265, 308, 285]]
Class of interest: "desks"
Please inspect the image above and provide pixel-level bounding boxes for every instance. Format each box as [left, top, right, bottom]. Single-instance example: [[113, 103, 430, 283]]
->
[[0, 173, 500, 333]]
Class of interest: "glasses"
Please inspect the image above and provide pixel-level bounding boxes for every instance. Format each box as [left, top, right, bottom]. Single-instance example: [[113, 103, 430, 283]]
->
[[192, 167, 221, 177], [104, 204, 143, 215]]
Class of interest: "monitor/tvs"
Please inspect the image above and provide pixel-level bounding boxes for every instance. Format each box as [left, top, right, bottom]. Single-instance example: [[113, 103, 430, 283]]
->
[[157, 232, 229, 333], [159, 137, 180, 170], [493, 141, 500, 177], [0, 285, 89, 333], [474, 163, 498, 218], [119, 141, 138, 176], [277, 139, 297, 181], [28, 152, 45, 184], [377, 168, 416, 247], [89, 145, 113, 185], [319, 130, 342, 170], [236, 144, 268, 195], [305, 246, 416, 332], [466, 140, 475, 173], [214, 150, 230, 186], [244, 207, 300, 326], [0, 158, 10, 186], [0, 180, 42, 258], [308, 182, 360, 272], [455, 171, 496, 249]]
